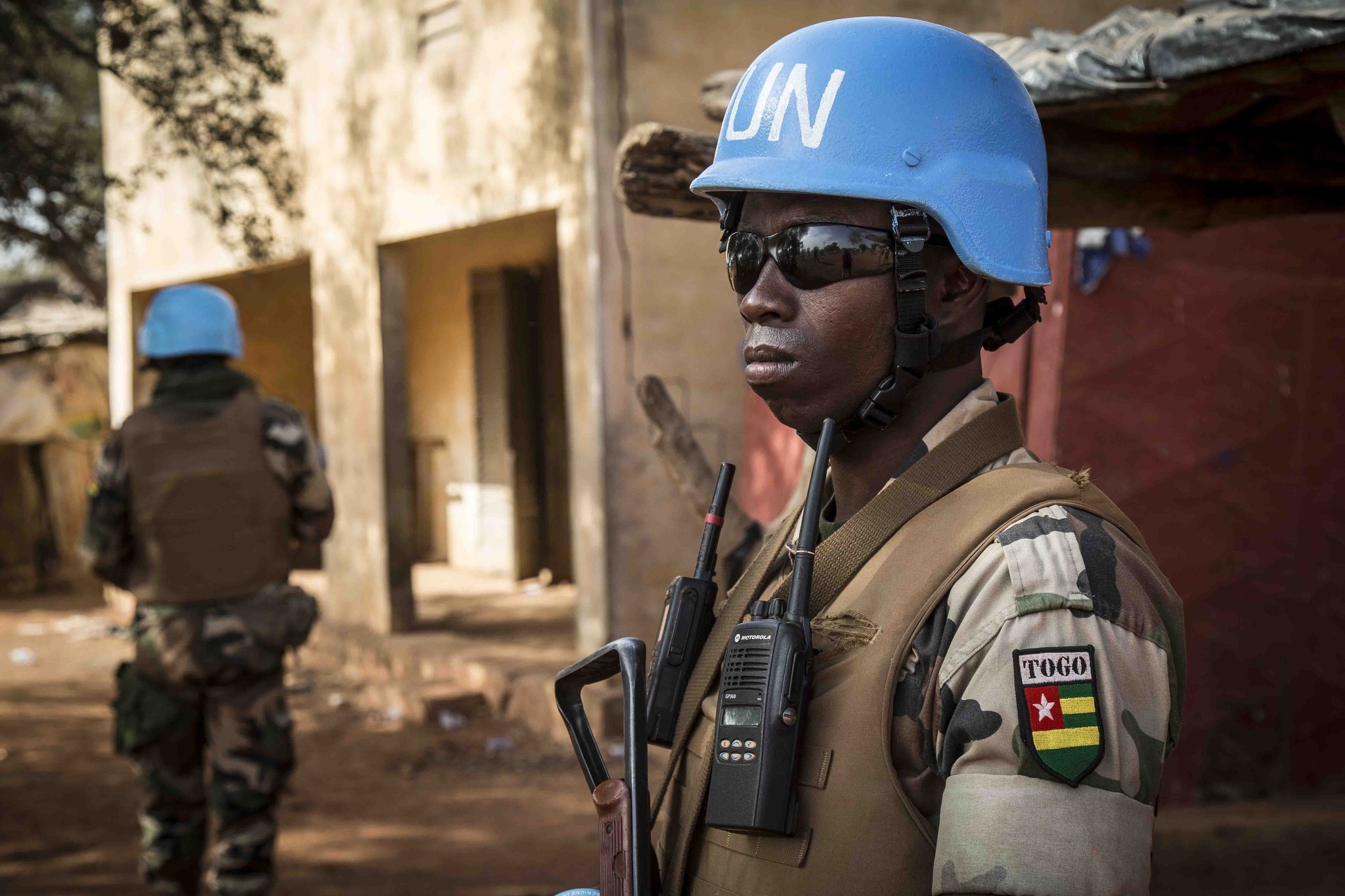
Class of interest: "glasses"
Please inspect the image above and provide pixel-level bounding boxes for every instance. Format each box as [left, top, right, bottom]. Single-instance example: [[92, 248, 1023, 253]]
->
[[717, 219, 953, 291]]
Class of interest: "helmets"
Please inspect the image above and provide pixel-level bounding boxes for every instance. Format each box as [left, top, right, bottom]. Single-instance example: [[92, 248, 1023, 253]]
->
[[687, 12, 1052, 290], [135, 281, 243, 363]]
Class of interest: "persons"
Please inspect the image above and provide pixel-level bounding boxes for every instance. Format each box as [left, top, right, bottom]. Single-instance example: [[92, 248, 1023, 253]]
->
[[659, 18, 1186, 895], [79, 283, 333, 895]]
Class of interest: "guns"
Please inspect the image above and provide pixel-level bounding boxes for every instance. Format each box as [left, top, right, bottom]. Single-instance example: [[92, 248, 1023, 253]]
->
[[553, 637, 672, 896]]
